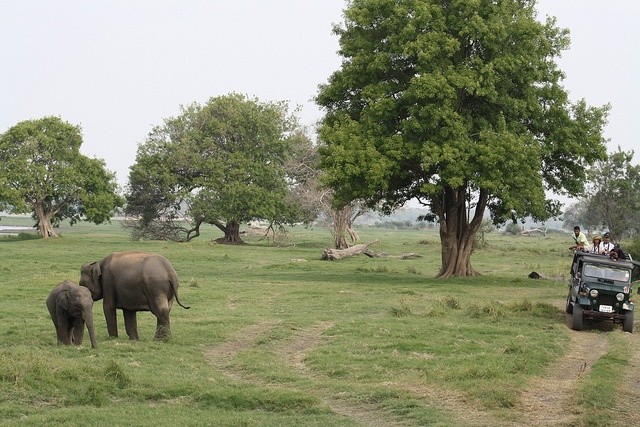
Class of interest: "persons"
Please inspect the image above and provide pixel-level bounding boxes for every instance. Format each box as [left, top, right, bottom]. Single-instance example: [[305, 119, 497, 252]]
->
[[577, 235, 605, 254], [569, 226, 592, 253], [610, 244, 626, 260], [600, 233, 615, 254]]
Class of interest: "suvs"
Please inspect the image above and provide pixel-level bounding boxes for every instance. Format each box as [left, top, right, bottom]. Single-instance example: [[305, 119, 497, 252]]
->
[[565, 252, 635, 334]]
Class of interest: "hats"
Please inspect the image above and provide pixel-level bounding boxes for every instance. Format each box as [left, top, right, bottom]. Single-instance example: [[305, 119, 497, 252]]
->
[[604, 233, 610, 238], [592, 235, 602, 243]]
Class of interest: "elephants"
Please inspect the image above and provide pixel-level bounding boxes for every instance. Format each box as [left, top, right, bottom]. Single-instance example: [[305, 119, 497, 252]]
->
[[79, 250, 191, 343], [46, 279, 98, 349]]
